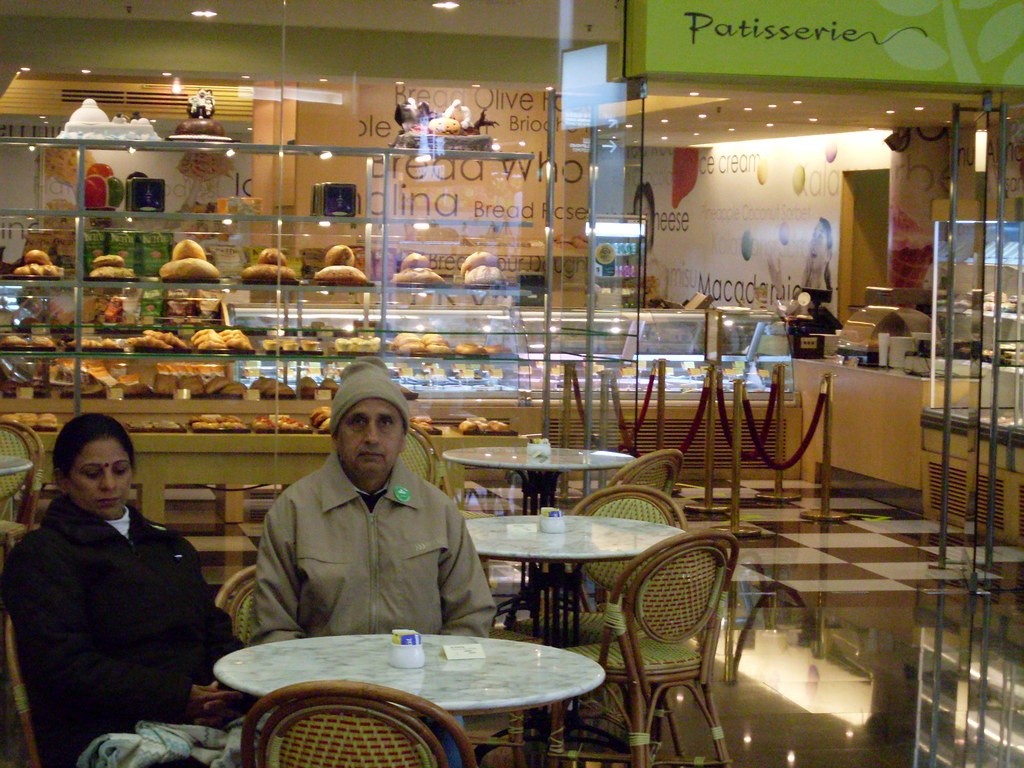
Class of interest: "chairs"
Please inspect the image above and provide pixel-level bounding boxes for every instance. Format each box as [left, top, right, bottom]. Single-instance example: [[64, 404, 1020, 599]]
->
[[241, 680, 477, 768], [505, 448, 739, 768], [401, 423, 452, 502], [4, 616, 42, 768], [213, 565, 255, 648], [0, 419, 45, 571]]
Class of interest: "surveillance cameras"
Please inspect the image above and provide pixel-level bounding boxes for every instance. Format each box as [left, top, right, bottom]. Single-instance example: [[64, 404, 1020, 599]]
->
[[884, 132, 902, 150]]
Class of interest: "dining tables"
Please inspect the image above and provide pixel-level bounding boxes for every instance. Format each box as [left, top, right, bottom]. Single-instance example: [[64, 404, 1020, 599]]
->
[[34, 426, 529, 523]]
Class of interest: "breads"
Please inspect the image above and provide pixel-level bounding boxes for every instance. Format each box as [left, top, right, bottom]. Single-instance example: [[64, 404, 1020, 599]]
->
[[953, 290, 1024, 313], [0, 238, 512, 434], [552, 234, 589, 249]]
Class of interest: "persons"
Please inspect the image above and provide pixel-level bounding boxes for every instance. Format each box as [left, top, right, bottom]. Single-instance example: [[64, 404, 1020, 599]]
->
[[5, 413, 303, 768], [252, 356, 498, 768], [764, 217, 834, 318], [626, 182, 667, 306]]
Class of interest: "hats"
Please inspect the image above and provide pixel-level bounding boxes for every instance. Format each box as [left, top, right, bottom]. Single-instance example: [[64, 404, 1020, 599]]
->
[[329, 355, 410, 436]]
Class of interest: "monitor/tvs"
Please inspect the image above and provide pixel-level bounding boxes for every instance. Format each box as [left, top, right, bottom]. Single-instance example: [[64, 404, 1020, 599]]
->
[[808, 306, 844, 330]]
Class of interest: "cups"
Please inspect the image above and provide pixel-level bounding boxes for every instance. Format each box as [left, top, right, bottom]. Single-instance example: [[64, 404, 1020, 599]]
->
[[388, 642, 424, 668], [526, 443, 551, 456], [539, 515, 565, 534]]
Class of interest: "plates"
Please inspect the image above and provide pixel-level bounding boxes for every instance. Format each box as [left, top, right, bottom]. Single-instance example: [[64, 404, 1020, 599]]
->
[[717, 305, 751, 313]]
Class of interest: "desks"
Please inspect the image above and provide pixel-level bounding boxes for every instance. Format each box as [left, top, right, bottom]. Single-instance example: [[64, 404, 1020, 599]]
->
[[442, 447, 637, 637], [464, 515, 691, 759], [0, 455, 34, 521], [212, 632, 605, 768]]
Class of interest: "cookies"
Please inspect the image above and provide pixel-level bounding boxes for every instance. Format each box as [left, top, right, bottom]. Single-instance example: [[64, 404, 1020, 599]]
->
[[22, 144, 232, 255]]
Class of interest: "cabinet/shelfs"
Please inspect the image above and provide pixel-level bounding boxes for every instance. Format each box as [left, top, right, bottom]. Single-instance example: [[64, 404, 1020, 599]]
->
[[0, 135, 535, 416]]
